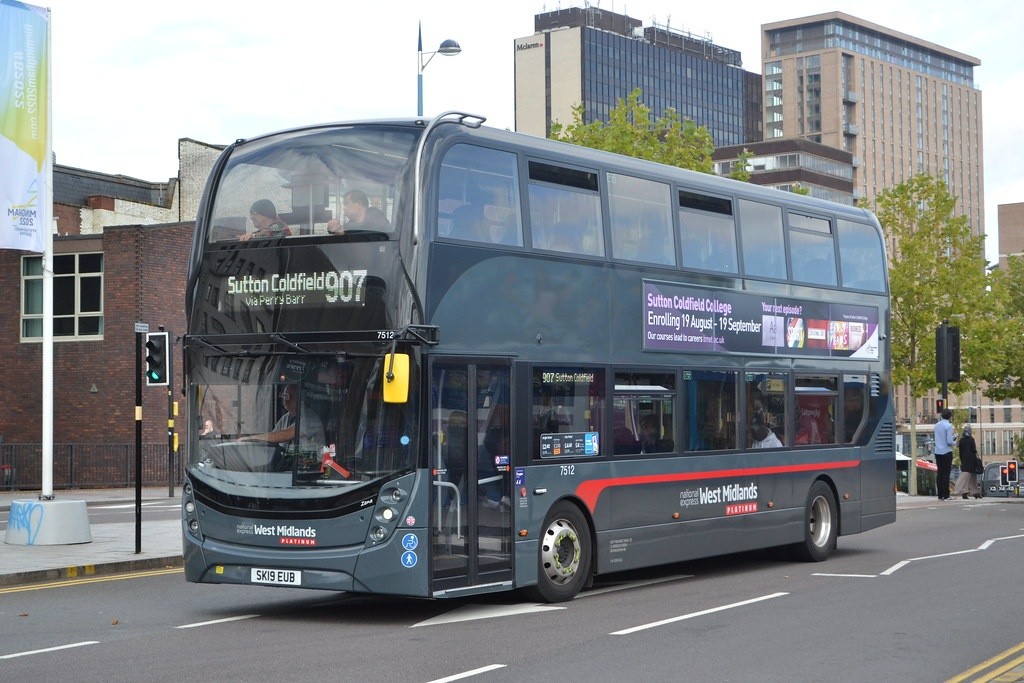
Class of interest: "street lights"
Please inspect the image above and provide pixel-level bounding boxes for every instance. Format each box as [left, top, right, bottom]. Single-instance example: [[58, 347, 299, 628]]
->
[[417, 20, 462, 116]]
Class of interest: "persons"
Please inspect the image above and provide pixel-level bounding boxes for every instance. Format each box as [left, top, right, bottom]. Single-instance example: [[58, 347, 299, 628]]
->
[[951, 422, 983, 500], [236, 382, 326, 454], [749, 414, 783, 449], [201, 419, 217, 435], [236, 199, 292, 242], [933, 408, 961, 503], [638, 413, 675, 453], [799, 376, 831, 445], [326, 189, 391, 234], [846, 388, 874, 445]]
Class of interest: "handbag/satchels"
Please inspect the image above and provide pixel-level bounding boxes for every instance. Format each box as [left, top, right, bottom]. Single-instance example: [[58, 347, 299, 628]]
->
[[974, 453, 983, 474]]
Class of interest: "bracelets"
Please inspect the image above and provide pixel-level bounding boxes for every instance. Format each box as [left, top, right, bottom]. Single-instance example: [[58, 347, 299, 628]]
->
[[250, 233, 257, 237]]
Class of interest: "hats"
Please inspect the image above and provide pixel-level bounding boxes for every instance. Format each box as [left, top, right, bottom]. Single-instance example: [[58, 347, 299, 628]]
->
[[939, 409, 953, 416], [252, 199, 277, 219]]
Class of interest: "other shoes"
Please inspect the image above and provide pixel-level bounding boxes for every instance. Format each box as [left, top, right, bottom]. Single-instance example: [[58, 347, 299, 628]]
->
[[482, 499, 499, 509], [962, 493, 969, 499], [974, 494, 983, 499], [444, 503, 458, 512], [499, 495, 511, 513], [947, 495, 956, 500], [938, 497, 948, 503]]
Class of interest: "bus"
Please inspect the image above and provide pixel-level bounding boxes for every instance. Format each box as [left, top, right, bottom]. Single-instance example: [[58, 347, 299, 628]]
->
[[895, 423, 1024, 492], [181, 112, 898, 603]]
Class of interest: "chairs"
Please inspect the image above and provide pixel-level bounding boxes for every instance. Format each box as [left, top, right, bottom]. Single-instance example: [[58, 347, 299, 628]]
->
[[312, 391, 339, 452], [448, 204, 860, 286]]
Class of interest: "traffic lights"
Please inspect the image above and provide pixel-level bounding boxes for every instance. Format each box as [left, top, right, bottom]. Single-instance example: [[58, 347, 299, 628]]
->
[[145, 332, 169, 386], [1006, 460, 1018, 482], [936, 400, 944, 415], [999, 466, 1010, 486]]
